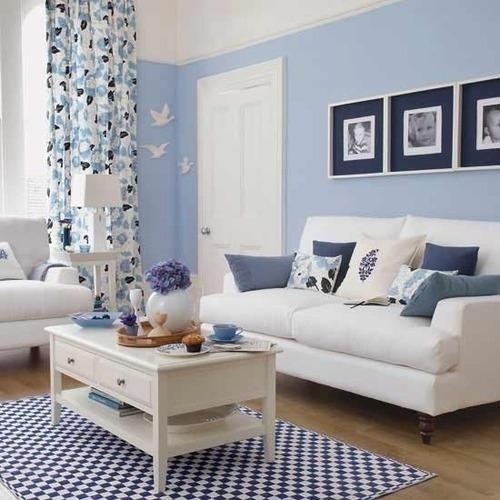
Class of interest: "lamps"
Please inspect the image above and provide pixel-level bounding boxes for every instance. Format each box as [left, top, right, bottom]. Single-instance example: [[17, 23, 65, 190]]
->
[[70, 173, 122, 252]]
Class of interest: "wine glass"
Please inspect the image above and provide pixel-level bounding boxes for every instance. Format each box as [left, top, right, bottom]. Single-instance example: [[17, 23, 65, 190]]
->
[[128, 288, 143, 323]]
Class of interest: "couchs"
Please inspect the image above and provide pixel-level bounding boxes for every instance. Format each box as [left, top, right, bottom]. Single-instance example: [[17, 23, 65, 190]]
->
[[198, 215, 500, 444]]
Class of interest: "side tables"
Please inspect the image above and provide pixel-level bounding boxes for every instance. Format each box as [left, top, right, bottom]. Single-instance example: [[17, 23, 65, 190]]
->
[[51, 250, 119, 313]]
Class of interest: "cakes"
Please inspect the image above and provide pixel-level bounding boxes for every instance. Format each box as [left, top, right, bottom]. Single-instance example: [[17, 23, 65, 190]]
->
[[181, 334, 205, 352]]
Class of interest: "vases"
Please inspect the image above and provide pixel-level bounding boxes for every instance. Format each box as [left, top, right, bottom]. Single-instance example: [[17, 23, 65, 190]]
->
[[146, 289, 193, 332]]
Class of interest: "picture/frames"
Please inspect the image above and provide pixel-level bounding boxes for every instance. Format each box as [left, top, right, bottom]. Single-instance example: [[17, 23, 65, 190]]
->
[[386, 83, 456, 176], [327, 95, 385, 180], [456, 74, 500, 172]]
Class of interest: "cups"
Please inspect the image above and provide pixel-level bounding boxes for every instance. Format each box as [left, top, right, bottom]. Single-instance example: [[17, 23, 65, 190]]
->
[[213, 323, 244, 340], [79, 245, 90, 253]]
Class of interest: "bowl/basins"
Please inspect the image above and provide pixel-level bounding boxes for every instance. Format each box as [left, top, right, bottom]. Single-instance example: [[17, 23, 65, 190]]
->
[[140, 404, 239, 433]]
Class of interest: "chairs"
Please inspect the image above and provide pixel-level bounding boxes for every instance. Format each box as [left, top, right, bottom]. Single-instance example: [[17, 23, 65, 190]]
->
[[0, 215, 92, 353]]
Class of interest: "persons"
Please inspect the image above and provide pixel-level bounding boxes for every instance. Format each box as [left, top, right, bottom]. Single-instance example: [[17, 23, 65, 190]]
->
[[346, 124, 369, 155], [409, 112, 436, 146], [483, 108, 500, 144]]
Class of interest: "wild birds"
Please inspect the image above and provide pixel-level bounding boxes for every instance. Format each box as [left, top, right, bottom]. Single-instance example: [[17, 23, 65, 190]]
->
[[177, 156, 194, 175], [140, 142, 171, 159], [151, 103, 175, 127]]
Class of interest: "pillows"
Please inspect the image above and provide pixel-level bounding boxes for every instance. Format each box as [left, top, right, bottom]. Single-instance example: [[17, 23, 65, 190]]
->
[[224, 253, 295, 292], [286, 250, 343, 295], [385, 264, 459, 306], [0, 242, 27, 280], [312, 241, 357, 292], [399, 272, 500, 318], [335, 234, 427, 302], [421, 243, 480, 276]]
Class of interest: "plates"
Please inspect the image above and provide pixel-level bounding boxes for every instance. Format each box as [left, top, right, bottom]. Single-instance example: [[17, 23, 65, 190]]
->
[[156, 343, 210, 356], [72, 311, 123, 327], [205, 334, 243, 343]]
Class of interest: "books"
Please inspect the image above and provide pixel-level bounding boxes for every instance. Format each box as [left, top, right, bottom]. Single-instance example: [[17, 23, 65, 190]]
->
[[86, 386, 145, 418], [344, 293, 392, 307]]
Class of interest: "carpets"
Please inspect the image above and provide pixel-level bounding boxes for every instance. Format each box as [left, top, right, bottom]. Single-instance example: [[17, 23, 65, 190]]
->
[[0, 394, 438, 500]]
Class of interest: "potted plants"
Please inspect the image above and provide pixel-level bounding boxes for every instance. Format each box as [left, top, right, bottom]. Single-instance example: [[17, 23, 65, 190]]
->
[[120, 309, 140, 336]]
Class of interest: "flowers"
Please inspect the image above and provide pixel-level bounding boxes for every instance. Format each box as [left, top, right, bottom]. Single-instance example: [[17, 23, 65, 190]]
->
[[144, 258, 192, 293]]
